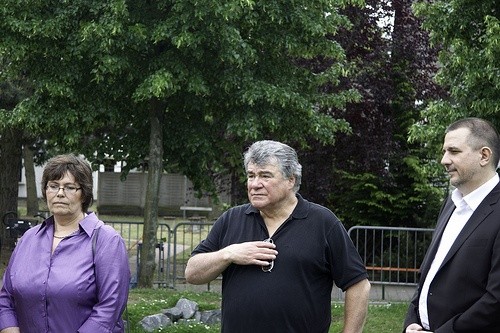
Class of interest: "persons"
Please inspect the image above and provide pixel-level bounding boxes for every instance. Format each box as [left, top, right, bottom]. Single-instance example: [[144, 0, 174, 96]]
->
[[185, 140, 372, 333], [0, 153, 129, 333], [402, 117, 500, 333]]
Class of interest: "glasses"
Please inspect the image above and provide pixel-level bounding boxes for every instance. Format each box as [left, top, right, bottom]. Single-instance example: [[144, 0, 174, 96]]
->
[[46, 182, 81, 194], [261, 237, 273, 272]]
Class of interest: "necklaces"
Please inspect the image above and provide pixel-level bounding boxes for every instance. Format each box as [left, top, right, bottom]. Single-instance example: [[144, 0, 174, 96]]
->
[[51, 213, 86, 239]]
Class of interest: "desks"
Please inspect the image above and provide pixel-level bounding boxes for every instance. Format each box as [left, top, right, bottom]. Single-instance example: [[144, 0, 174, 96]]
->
[[180, 205, 212, 234]]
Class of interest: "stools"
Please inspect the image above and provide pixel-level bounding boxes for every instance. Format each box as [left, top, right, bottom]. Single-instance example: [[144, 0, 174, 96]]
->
[[136, 238, 164, 286]]
